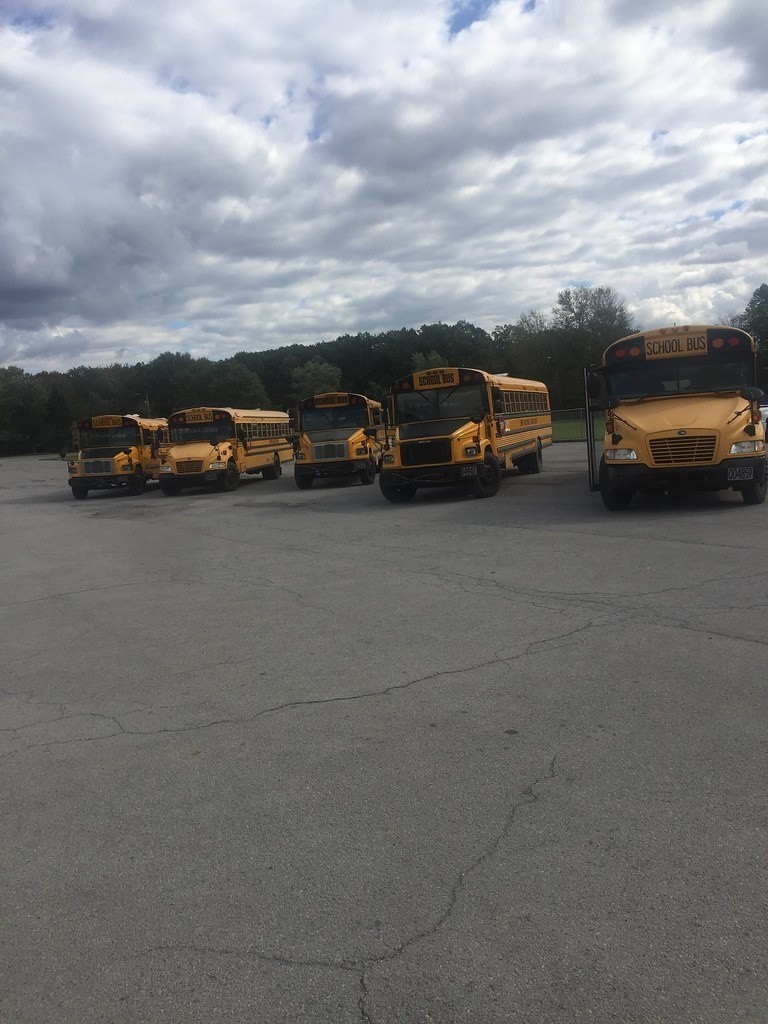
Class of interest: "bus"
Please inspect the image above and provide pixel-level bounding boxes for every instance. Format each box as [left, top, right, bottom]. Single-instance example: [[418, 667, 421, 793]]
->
[[584, 324, 767, 511], [286, 392, 390, 488], [378, 367, 554, 503], [150, 405, 293, 496], [58, 413, 169, 498]]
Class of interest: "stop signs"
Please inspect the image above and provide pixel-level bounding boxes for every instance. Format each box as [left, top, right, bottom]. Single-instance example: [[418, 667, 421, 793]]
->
[[498, 413, 505, 435]]
